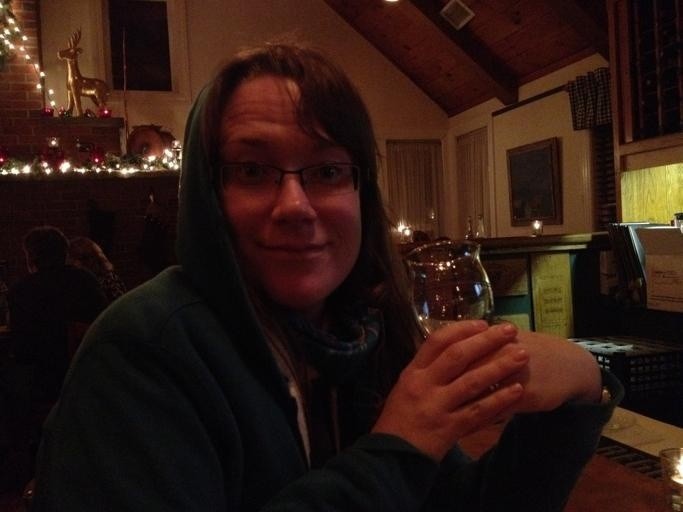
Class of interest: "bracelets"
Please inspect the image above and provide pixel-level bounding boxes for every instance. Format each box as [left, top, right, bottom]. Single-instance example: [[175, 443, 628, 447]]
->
[[600, 385, 611, 407]]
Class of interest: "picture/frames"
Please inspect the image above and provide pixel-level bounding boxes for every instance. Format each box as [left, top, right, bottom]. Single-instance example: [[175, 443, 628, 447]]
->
[[504, 136, 563, 225]]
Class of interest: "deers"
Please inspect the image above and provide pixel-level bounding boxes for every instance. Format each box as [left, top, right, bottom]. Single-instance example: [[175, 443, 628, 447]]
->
[[56, 26, 111, 117]]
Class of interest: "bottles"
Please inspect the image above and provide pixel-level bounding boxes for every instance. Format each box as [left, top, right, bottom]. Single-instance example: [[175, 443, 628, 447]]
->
[[463, 215, 473, 240], [474, 214, 487, 240]]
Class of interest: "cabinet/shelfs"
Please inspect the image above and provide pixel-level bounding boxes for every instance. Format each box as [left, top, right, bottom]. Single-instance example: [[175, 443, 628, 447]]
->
[[604, 0, 683, 226]]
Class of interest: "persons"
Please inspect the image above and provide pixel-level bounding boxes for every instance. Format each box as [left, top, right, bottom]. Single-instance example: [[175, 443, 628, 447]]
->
[[67, 236, 125, 304], [32, 41, 623, 506], [6, 227, 107, 405]]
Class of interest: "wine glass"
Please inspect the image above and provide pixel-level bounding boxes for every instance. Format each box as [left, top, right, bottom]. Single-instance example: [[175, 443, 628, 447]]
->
[[402, 238, 512, 434]]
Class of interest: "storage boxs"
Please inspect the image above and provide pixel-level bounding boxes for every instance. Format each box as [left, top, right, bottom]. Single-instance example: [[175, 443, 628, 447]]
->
[[632, 226, 682, 315]]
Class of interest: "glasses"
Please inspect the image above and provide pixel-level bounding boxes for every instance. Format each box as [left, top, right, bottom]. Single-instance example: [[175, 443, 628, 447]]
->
[[220, 157, 358, 196]]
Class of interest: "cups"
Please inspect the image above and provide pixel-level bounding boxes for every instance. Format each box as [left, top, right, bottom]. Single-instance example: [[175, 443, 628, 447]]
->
[[674, 213, 683, 227], [657, 444, 683, 512]]
